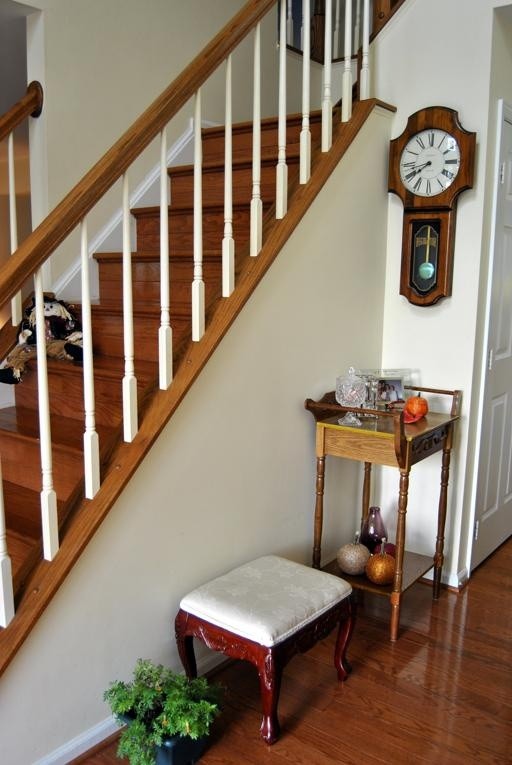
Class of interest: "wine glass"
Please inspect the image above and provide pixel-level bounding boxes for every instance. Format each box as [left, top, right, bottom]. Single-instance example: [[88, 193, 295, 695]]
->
[[335, 366, 367, 427]]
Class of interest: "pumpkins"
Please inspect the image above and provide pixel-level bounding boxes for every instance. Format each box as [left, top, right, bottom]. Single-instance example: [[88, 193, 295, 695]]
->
[[405, 392, 428, 418]]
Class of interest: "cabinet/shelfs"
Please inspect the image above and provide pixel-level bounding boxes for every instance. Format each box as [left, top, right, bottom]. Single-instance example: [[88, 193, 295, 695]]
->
[[303, 383, 463, 644]]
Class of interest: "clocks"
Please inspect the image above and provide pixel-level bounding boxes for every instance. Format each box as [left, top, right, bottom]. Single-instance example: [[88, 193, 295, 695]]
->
[[387, 106, 477, 308]]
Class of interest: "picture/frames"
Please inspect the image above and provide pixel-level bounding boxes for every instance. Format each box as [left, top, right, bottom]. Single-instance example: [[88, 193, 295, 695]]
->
[[375, 377, 405, 407], [276, 1, 326, 66], [331, 0, 364, 63]]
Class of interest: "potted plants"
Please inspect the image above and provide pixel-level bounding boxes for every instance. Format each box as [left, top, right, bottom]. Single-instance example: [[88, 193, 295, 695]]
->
[[102, 658, 224, 765]]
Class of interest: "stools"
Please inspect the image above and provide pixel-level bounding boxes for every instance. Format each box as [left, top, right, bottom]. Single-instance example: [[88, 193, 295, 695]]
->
[[174, 554, 357, 747]]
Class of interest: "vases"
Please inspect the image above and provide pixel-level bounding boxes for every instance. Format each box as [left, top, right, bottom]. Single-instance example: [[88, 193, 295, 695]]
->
[[361, 506, 389, 543]]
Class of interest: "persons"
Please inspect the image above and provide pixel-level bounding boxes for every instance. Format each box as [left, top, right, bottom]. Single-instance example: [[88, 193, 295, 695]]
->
[[1, 290, 84, 384]]
